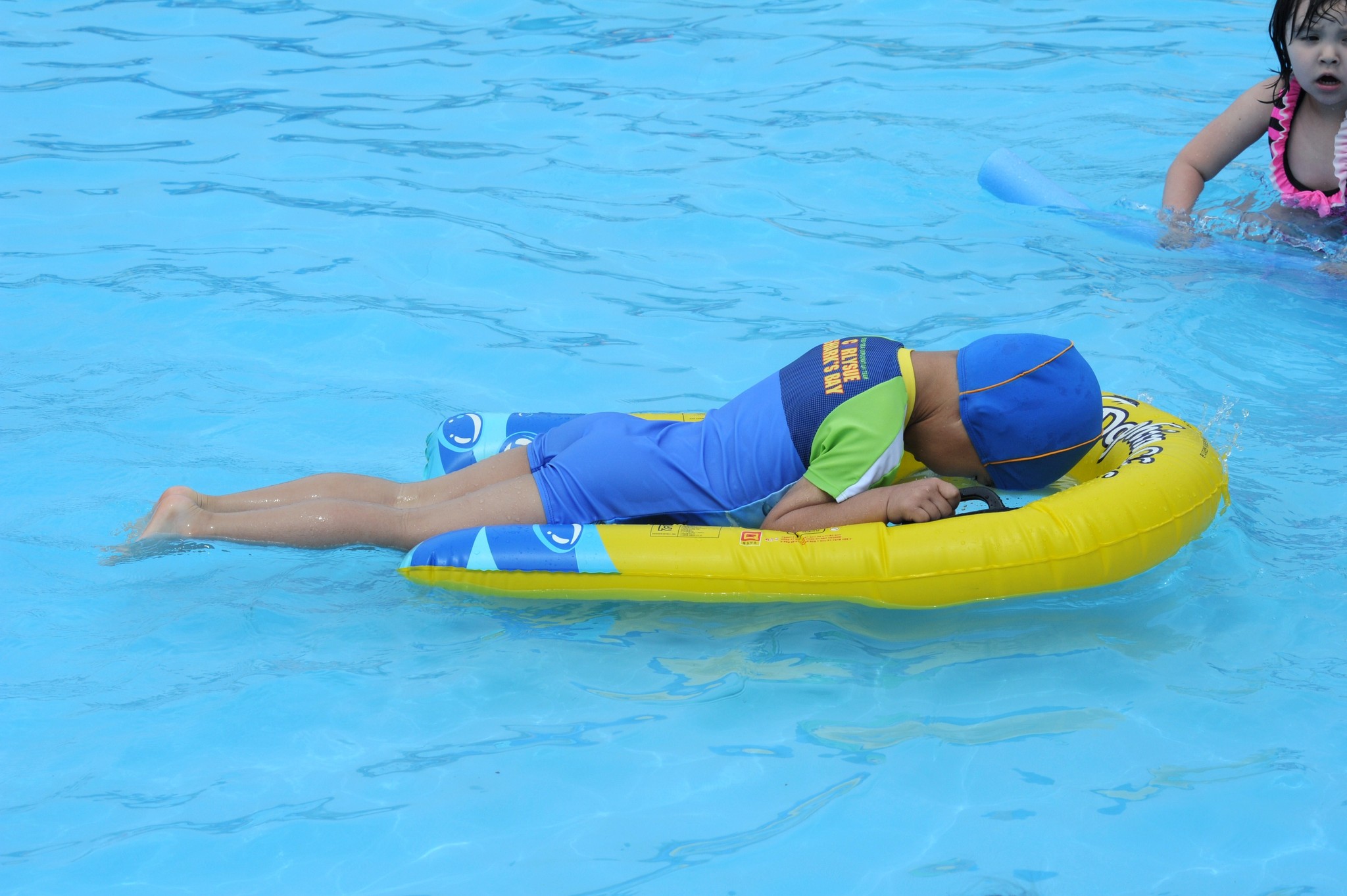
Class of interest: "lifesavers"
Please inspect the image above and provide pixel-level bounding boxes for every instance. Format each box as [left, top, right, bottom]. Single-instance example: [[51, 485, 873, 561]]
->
[[395, 390, 1226, 609]]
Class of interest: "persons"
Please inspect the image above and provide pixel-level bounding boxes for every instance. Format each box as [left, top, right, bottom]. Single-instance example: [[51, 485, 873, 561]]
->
[[117, 335, 1103, 556], [1155, 0, 1347, 278]]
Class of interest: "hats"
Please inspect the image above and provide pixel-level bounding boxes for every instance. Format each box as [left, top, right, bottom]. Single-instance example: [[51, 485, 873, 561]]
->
[[958, 332, 1104, 490]]
